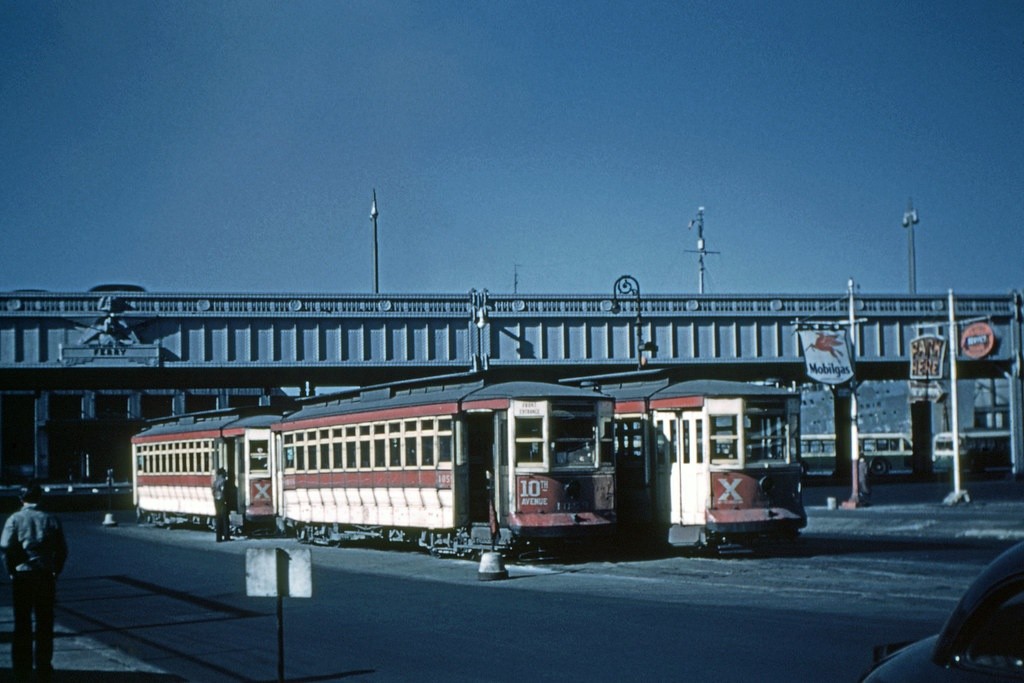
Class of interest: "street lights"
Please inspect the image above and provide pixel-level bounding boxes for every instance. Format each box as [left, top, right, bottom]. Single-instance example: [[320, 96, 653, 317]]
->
[[611, 275, 645, 372], [688, 207, 706, 295], [902, 194, 920, 295], [368, 184, 378, 294]]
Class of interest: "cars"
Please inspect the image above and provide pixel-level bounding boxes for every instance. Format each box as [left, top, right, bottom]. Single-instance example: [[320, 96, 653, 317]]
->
[[859, 542, 1024, 683]]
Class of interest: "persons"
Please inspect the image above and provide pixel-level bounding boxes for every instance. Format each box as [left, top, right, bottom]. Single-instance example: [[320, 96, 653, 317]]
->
[[858, 449, 870, 506], [211, 467, 235, 542], [0, 481, 69, 683]]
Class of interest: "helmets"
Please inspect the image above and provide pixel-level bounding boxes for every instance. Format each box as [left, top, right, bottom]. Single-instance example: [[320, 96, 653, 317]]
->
[[18, 478, 42, 504], [216, 468, 225, 475]]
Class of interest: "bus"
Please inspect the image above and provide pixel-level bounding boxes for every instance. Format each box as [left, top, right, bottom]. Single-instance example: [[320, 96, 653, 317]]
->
[[129, 373, 621, 564], [931, 431, 1012, 475], [783, 433, 913, 476], [556, 369, 809, 550]]
